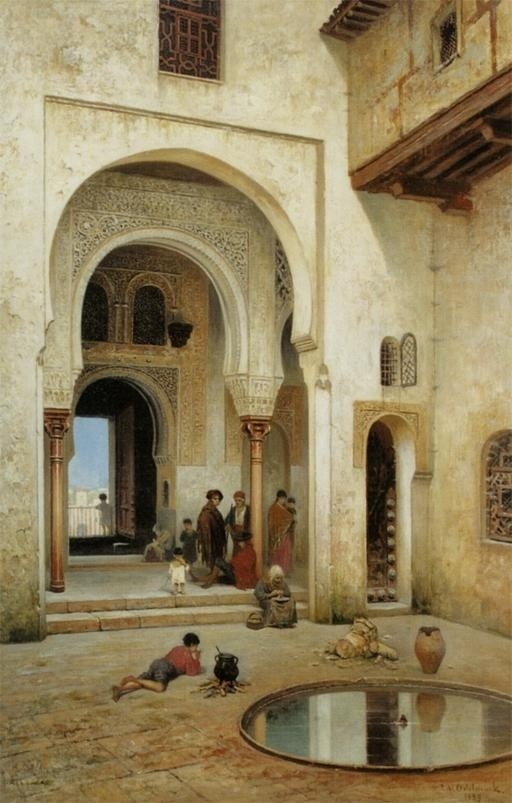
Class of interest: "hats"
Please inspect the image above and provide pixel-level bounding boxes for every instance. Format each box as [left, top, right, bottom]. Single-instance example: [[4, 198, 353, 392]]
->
[[234, 491, 244, 498], [207, 490, 222, 499], [234, 531, 251, 540]]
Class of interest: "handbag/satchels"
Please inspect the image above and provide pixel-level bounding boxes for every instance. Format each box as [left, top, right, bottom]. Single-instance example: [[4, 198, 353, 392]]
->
[[247, 613, 264, 630]]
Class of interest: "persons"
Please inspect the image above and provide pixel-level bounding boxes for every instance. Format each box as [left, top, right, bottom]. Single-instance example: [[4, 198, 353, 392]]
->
[[184, 529, 257, 590], [221, 490, 250, 559], [179, 517, 199, 570], [110, 631, 202, 702], [268, 488, 299, 576], [141, 521, 174, 564], [250, 564, 297, 630], [283, 497, 298, 522], [167, 546, 191, 594], [195, 489, 228, 568], [95, 492, 114, 536]]
